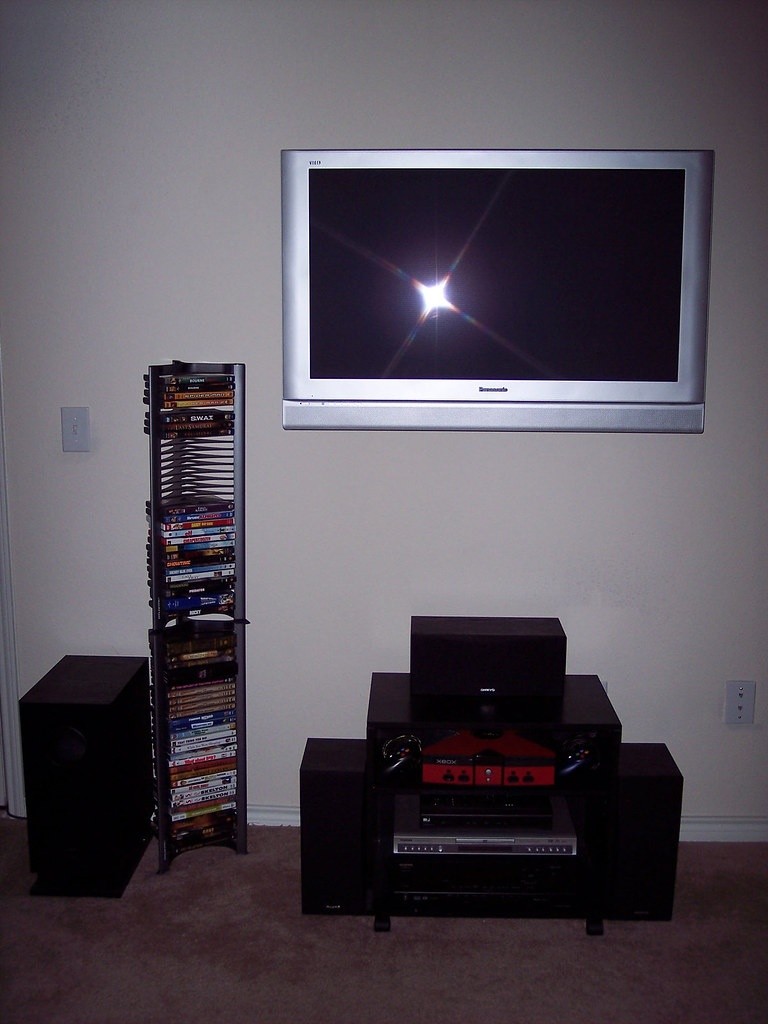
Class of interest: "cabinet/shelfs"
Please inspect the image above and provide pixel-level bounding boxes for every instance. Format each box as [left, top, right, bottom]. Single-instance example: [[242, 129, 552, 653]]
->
[[301, 736, 683, 927], [149, 363, 249, 876]]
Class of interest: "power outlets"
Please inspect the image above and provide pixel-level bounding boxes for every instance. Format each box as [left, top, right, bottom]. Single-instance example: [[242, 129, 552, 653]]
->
[[727, 681, 757, 724]]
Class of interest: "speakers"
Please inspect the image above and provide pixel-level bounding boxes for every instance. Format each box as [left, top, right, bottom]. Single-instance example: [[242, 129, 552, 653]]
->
[[17, 655, 150, 896], [301, 619, 683, 922]]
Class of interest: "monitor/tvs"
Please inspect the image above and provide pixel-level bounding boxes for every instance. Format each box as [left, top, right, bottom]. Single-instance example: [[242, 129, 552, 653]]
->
[[278, 148, 715, 434]]
